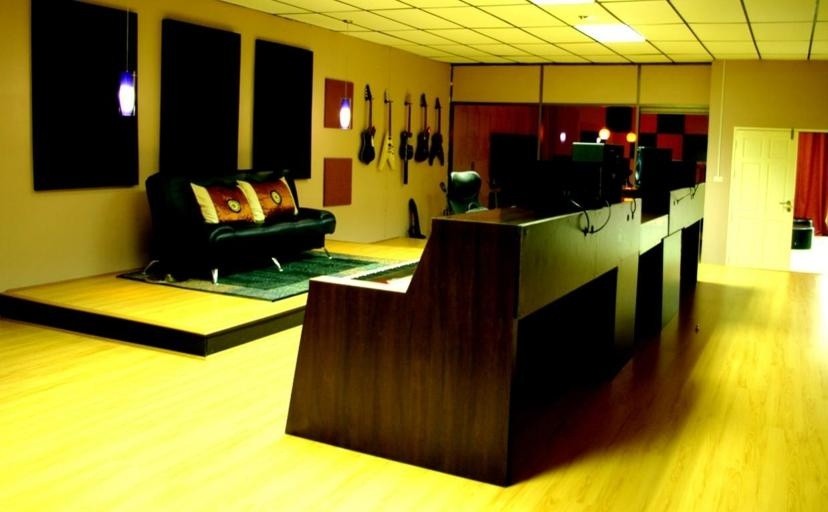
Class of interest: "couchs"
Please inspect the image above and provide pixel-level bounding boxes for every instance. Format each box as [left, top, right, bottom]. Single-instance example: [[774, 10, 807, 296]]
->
[[143, 167, 336, 284]]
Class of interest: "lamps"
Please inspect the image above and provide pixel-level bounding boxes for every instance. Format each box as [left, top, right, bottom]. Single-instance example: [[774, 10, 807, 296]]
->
[[339, 18, 352, 131]]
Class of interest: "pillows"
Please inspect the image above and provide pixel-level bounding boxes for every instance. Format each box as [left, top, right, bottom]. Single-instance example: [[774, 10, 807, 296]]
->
[[236, 179, 298, 224], [190, 181, 265, 224]]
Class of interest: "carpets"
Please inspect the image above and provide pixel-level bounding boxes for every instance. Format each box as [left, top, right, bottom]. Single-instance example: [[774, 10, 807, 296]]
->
[[114, 249, 389, 302]]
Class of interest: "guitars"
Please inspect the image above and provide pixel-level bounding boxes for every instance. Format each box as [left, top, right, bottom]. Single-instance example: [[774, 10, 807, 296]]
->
[[379, 88, 395, 170], [360, 84, 375, 164], [400, 102, 413, 160], [415, 94, 429, 162], [429, 97, 444, 166]]
